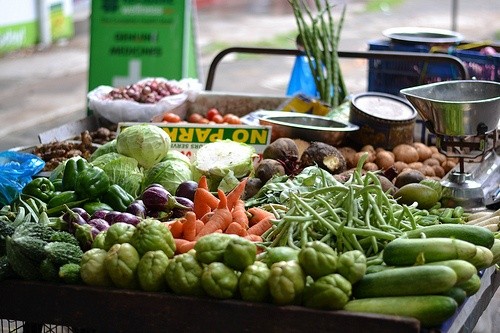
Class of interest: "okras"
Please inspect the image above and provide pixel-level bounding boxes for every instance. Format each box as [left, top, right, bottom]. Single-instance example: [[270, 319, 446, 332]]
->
[[0, 193, 59, 228]]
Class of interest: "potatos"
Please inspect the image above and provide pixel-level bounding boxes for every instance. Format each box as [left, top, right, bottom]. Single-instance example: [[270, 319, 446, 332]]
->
[[338, 142, 456, 181]]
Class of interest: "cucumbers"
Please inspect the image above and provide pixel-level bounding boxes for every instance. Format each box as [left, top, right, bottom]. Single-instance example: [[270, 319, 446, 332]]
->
[[344, 223, 500, 323], [0, 215, 83, 285]]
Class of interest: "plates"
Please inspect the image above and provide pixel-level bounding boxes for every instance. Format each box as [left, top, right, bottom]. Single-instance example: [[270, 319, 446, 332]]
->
[[382, 28, 465, 46]]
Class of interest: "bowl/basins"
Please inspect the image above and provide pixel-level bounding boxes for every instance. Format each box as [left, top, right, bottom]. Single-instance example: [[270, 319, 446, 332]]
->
[[258, 114, 359, 148]]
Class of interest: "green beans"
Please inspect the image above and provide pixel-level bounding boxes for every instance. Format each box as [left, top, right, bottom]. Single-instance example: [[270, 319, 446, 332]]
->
[[287, 0, 347, 107], [252, 152, 422, 267]]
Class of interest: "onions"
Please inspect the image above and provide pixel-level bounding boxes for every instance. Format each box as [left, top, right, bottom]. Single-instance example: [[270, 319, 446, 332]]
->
[[105, 80, 182, 104]]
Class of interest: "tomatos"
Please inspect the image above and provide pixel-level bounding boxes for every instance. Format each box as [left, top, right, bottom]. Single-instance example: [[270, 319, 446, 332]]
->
[[160, 108, 242, 125]]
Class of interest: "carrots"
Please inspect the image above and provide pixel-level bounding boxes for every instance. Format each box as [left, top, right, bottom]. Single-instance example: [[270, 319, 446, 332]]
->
[[167, 175, 276, 253]]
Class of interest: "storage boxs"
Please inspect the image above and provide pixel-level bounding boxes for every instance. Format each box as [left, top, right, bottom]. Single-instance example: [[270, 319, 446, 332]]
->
[[152, 91, 291, 165]]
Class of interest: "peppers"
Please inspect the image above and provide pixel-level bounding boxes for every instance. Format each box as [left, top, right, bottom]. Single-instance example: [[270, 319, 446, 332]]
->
[[418, 200, 466, 226], [22, 159, 134, 215]]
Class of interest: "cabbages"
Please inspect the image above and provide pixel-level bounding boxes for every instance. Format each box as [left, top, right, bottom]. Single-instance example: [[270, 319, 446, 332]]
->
[[88, 125, 260, 198]]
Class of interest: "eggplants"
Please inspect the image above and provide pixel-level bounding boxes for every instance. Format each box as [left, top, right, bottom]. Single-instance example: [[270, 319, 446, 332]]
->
[[46, 180, 199, 252]]
